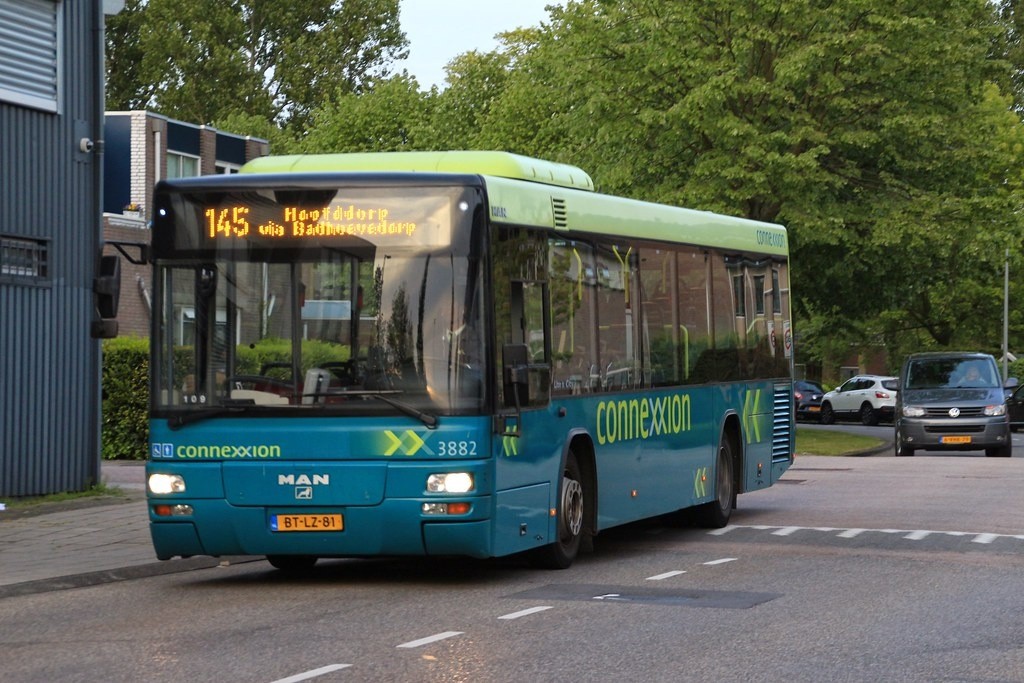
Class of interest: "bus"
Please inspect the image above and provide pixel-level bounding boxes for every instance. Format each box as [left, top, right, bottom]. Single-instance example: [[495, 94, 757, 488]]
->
[[89, 149, 797, 572]]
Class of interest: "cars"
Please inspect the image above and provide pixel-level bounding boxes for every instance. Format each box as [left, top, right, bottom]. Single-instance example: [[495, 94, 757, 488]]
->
[[794, 379, 825, 421], [1004, 381, 1024, 434]]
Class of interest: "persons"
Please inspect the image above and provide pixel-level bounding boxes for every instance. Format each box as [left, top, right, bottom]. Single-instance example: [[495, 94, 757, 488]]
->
[[386, 288, 483, 398], [958, 365, 987, 386]]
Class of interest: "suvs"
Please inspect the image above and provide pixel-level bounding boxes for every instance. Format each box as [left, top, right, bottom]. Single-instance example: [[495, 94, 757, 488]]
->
[[818, 373, 900, 426]]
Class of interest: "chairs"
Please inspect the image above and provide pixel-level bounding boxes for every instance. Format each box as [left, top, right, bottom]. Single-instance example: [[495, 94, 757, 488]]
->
[[320, 362, 356, 386], [914, 366, 935, 385], [529, 276, 752, 398], [257, 361, 303, 399]]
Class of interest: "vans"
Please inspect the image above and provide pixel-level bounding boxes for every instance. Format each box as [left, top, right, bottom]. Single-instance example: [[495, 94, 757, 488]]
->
[[886, 350, 1021, 459]]
